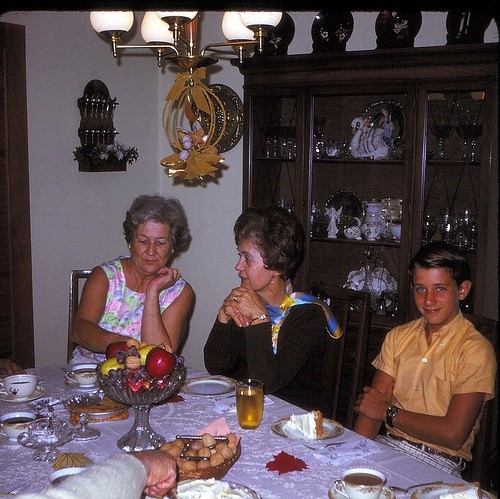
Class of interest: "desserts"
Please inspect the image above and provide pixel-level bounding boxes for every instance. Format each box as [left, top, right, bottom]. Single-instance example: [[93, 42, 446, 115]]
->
[[289, 411, 324, 439]]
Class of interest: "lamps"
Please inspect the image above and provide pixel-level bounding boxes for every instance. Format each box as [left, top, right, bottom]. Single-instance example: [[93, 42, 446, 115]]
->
[[90, 11, 283, 188]]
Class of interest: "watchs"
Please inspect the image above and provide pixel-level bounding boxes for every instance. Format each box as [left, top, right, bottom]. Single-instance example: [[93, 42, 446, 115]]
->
[[386, 406, 399, 427]]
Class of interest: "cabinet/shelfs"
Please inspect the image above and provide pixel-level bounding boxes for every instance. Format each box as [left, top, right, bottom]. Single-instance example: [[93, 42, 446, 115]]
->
[[229, 45, 500, 384]]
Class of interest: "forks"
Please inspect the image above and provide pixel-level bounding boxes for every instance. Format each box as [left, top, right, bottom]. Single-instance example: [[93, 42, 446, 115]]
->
[[305, 441, 347, 450]]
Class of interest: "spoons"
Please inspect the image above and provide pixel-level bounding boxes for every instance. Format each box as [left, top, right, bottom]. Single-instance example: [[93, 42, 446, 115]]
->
[[389, 481, 443, 494]]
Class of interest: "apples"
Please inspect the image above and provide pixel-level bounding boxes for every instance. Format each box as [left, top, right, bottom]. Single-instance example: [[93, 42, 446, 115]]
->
[[101, 341, 177, 376]]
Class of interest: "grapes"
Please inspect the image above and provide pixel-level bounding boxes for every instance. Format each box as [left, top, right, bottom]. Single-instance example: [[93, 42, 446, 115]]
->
[[97, 345, 185, 391]]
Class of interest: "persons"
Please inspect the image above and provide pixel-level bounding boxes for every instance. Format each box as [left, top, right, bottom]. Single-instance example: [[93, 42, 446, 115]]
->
[[203, 208, 343, 419], [69, 194, 195, 364], [0, 449, 176, 499], [0, 359, 26, 376], [353, 240, 497, 481], [326, 207, 339, 236], [379, 107, 393, 142]]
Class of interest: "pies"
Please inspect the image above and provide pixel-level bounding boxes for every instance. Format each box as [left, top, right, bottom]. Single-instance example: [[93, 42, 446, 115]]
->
[[69, 395, 128, 412]]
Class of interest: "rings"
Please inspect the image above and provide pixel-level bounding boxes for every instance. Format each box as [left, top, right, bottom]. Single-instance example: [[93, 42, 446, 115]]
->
[[233, 297, 237, 301]]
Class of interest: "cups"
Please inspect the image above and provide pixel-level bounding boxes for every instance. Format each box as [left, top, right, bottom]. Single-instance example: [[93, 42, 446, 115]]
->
[[48, 467, 85, 487], [0, 374, 37, 399], [0, 412, 37, 441], [389, 224, 401, 241], [236, 379, 264, 429], [65, 364, 98, 387], [334, 468, 385, 499]]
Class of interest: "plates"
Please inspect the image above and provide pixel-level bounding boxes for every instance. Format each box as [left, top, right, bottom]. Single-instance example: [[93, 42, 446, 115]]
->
[[271, 417, 344, 440], [254, 8, 495, 41], [410, 483, 495, 499], [328, 485, 395, 499], [63, 392, 132, 419], [343, 266, 398, 311], [357, 101, 406, 143], [326, 189, 364, 222], [178, 375, 238, 398], [0, 386, 47, 402], [64, 377, 101, 392], [145, 479, 262, 499]]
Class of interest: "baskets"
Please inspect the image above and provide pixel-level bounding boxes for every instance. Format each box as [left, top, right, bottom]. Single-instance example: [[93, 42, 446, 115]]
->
[[177, 442, 241, 481]]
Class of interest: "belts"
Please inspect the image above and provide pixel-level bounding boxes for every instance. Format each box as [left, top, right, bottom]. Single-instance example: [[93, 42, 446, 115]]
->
[[389, 433, 465, 467]]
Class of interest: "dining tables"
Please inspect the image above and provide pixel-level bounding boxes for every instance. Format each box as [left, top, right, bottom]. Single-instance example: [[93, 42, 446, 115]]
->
[[0, 368, 498, 499]]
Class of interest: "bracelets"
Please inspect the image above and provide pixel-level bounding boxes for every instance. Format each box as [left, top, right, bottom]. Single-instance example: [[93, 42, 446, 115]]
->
[[250, 313, 270, 325]]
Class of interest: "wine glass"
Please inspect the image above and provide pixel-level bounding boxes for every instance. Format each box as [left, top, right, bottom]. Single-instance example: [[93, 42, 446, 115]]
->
[[429, 113, 483, 162], [423, 206, 477, 250], [261, 115, 327, 158]]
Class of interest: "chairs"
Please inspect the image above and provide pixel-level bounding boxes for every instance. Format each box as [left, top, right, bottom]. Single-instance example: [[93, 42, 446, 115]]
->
[[297, 284, 370, 432], [457, 313, 500, 495], [67, 270, 91, 364]]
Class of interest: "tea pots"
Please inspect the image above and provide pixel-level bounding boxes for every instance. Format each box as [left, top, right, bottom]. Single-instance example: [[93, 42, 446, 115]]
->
[[354, 197, 386, 240]]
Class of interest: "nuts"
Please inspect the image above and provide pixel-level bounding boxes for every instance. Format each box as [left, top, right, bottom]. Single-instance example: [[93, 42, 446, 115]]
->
[[125, 338, 140, 348], [126, 356, 141, 369], [159, 433, 238, 470], [158, 343, 172, 353]]
